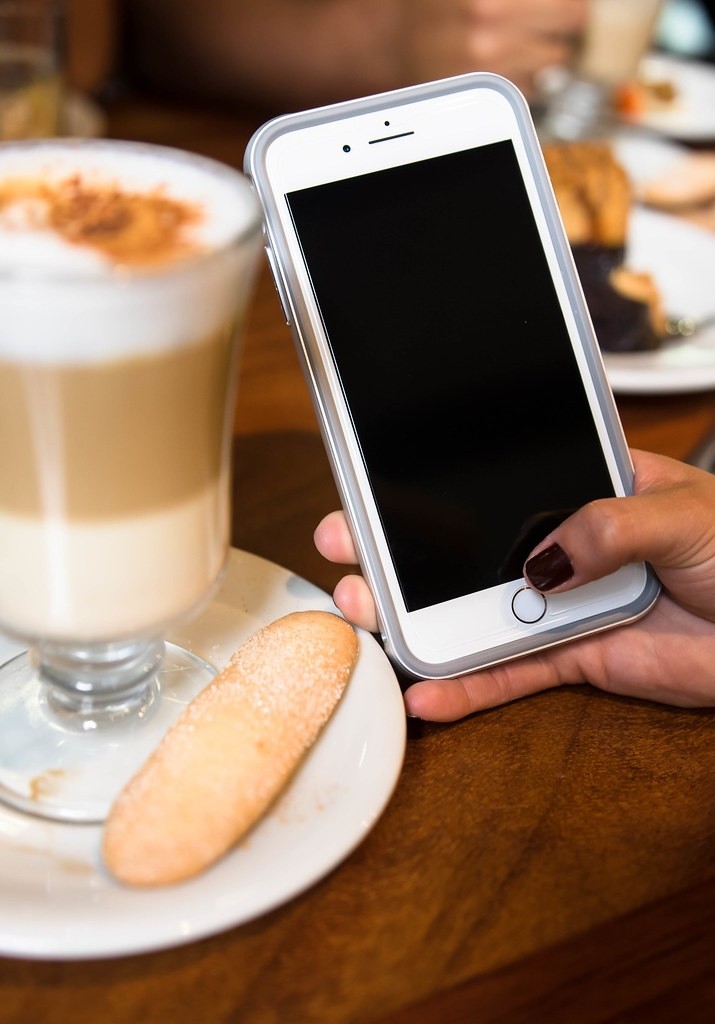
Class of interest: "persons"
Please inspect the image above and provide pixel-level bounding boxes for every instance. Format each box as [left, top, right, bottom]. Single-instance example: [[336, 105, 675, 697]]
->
[[313, 448, 715, 723]]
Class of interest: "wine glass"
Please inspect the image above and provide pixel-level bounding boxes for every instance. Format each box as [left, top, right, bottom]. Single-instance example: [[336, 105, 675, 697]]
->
[[0, 140, 269, 826]]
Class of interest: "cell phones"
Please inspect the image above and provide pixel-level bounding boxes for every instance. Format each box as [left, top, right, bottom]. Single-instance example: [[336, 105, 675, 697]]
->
[[243, 73, 665, 683]]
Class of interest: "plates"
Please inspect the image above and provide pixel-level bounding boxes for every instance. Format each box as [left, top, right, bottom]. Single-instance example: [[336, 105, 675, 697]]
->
[[0, 546, 406, 961], [600, 203, 715, 394]]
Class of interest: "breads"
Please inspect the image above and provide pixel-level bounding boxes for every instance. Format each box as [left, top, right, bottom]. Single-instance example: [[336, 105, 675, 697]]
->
[[100, 610, 358, 888]]
